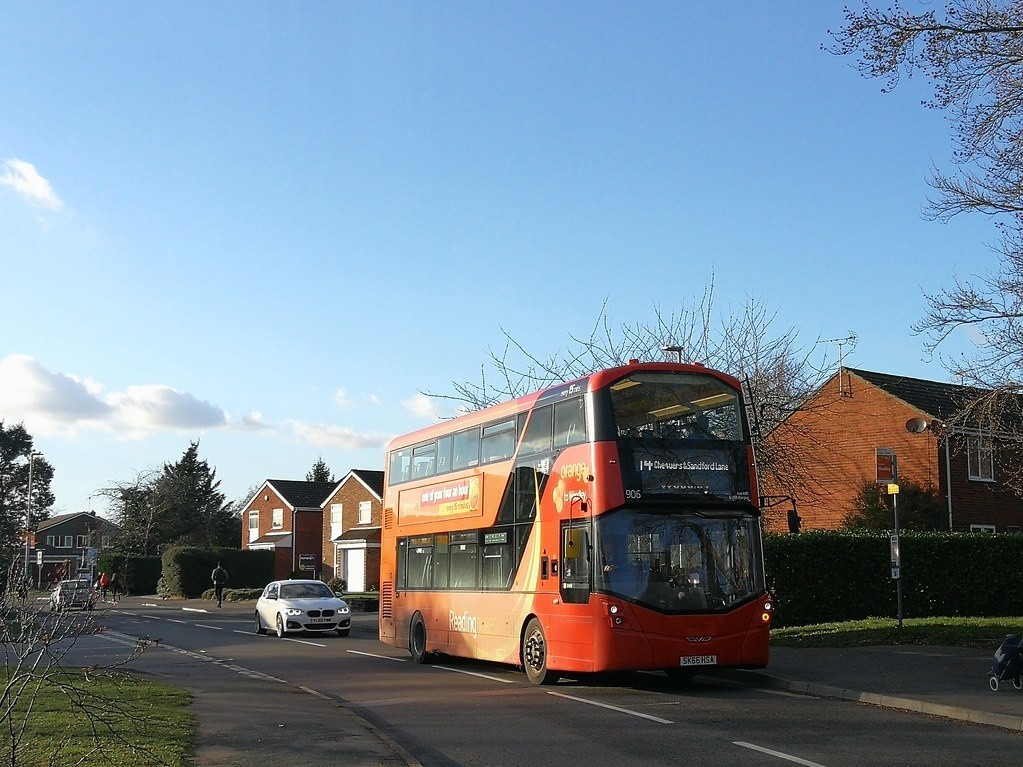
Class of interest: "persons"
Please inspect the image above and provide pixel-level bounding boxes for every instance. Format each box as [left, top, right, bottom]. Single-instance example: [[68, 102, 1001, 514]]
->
[[99, 573, 108, 602], [96, 572, 102, 591], [688, 416, 716, 439], [109, 573, 123, 602], [210, 561, 229, 609], [602, 536, 635, 571]]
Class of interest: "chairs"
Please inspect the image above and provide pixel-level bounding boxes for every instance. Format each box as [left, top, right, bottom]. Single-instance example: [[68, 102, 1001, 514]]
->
[[401, 454, 468, 482], [663, 425, 682, 439], [642, 430, 656, 437]]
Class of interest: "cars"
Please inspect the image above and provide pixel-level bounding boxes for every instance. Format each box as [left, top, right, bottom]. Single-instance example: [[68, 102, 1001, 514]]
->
[[255, 578, 351, 638], [50, 579, 93, 612]]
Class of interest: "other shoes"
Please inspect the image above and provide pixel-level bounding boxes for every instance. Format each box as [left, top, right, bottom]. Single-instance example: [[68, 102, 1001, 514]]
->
[[217, 601, 221, 609]]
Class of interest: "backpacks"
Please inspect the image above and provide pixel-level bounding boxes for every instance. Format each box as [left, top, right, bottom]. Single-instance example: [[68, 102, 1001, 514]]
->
[[215, 568, 226, 583]]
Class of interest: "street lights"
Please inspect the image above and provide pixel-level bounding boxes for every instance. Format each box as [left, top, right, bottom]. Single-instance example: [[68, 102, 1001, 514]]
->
[[662, 345, 686, 568], [24, 453, 43, 577]]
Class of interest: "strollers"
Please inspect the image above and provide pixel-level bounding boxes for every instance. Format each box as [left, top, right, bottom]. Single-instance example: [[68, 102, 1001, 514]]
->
[[987, 634, 1023, 691]]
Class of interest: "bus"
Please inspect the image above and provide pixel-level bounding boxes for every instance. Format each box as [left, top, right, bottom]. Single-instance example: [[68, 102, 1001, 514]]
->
[[378, 358, 803, 686]]
